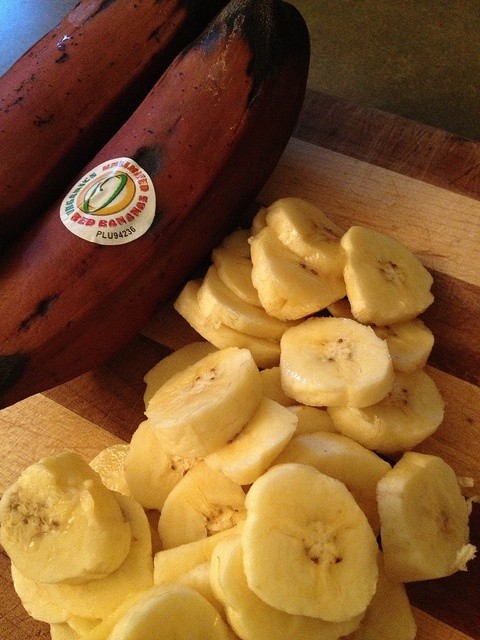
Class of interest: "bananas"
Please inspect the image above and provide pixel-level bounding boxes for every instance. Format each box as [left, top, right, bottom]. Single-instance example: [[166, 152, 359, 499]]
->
[[2, 1, 313, 408], [2, 0, 220, 250]]
